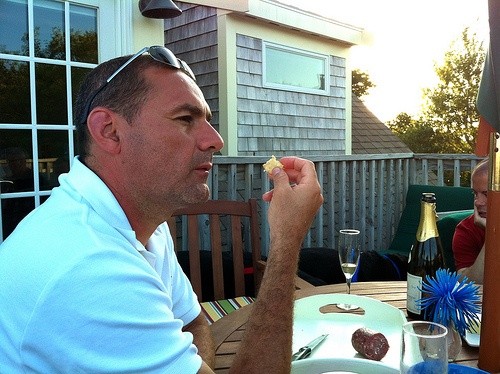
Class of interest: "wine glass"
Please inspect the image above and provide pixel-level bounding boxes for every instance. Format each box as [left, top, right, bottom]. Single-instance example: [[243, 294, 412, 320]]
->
[[336, 229, 360, 311]]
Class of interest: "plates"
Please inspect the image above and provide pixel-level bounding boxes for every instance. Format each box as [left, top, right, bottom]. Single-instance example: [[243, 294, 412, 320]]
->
[[457, 284, 484, 347]]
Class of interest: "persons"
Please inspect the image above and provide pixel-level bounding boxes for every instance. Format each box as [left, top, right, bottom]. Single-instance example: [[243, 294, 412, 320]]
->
[[451, 156, 493, 285], [0, 44, 324, 373]]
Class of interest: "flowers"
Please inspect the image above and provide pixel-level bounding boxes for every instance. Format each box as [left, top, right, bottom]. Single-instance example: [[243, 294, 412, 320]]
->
[[415, 268, 482, 331]]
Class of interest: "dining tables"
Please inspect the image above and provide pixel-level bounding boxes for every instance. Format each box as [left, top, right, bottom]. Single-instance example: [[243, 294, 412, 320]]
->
[[208, 280, 491, 373]]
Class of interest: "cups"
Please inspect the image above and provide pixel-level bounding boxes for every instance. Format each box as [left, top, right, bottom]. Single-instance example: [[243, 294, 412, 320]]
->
[[400, 321, 449, 374]]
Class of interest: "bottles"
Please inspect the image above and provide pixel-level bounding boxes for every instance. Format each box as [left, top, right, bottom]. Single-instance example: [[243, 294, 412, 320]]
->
[[407, 193, 446, 320]]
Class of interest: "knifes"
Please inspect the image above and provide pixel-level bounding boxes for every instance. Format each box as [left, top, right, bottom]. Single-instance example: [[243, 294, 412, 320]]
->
[[291, 334, 328, 362]]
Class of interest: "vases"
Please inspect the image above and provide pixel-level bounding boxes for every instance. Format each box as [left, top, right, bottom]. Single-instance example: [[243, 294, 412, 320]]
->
[[418, 320, 463, 364]]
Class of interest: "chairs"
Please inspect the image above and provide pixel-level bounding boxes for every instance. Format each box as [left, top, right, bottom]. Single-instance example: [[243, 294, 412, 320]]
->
[[383, 184, 475, 265], [167, 198, 314, 307]]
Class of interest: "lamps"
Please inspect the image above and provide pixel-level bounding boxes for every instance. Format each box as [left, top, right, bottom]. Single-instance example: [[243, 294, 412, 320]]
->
[[139, 0, 182, 20]]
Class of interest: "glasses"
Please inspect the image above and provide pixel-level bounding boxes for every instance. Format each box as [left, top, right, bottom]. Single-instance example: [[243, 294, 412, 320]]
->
[[80, 46, 196, 124]]
